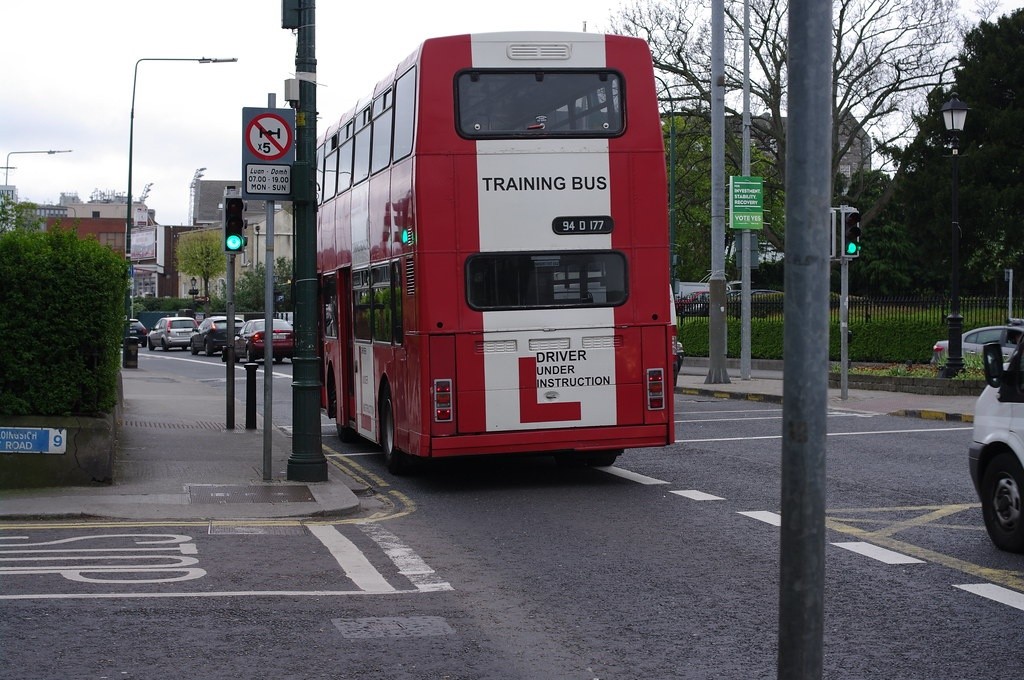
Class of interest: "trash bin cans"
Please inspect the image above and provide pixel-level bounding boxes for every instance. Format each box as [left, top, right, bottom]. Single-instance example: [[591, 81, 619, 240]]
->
[[122, 335, 139, 368]]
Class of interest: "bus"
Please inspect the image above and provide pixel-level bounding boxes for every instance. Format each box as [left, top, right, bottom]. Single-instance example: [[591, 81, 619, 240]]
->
[[315, 32, 684, 478]]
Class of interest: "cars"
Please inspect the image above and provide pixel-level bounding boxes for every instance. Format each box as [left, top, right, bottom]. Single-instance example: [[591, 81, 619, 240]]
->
[[189, 316, 246, 356], [675, 289, 779, 313], [121, 319, 148, 347], [969, 332, 1024, 555], [234, 319, 294, 364], [147, 317, 200, 351], [930, 324, 1024, 366]]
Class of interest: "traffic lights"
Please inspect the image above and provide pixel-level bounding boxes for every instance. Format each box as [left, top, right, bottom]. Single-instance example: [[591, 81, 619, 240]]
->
[[844, 212, 859, 255], [225, 198, 243, 253]]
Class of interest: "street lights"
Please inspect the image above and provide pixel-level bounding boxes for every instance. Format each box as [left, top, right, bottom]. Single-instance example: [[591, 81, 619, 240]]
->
[[938, 92, 972, 380], [123, 57, 238, 371], [191, 277, 197, 303], [5, 150, 72, 186]]
